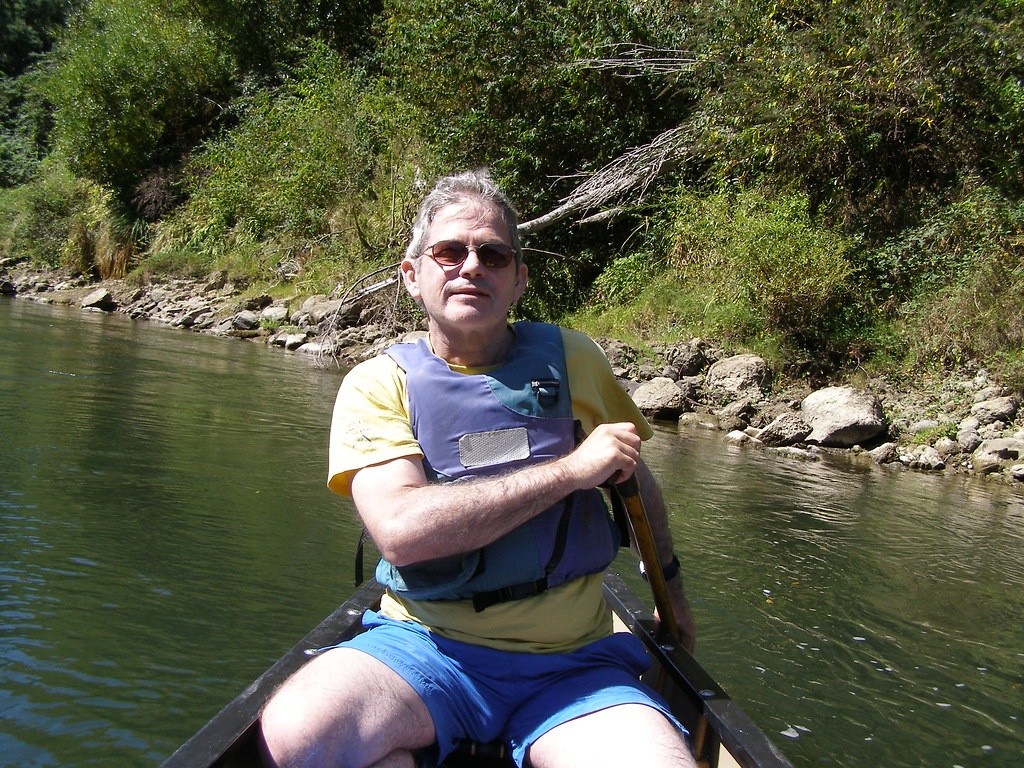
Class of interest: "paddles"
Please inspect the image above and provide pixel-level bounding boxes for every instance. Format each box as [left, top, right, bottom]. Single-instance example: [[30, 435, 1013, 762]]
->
[[606, 466, 683, 644]]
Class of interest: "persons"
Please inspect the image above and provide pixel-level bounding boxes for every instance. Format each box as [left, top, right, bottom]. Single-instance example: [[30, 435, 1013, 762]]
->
[[259, 169, 699, 768]]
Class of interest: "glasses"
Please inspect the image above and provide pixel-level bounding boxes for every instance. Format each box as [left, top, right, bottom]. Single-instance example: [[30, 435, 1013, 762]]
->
[[417, 240, 517, 268]]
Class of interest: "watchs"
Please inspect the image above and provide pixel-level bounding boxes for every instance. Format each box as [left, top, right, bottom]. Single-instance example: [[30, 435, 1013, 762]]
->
[[639, 557, 680, 582]]
